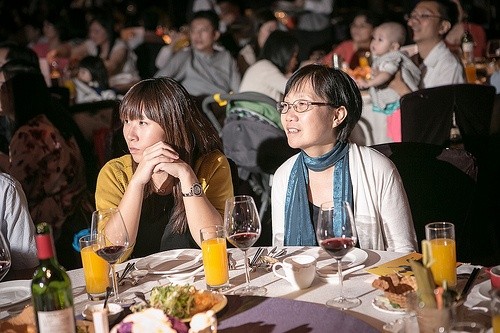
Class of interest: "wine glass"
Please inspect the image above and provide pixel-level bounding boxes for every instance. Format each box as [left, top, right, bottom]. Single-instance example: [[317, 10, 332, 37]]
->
[[316, 202, 361, 310], [223, 196, 268, 296], [90, 208, 130, 307]]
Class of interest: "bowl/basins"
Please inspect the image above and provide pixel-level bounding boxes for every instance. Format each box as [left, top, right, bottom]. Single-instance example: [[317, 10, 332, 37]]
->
[[82, 304, 122, 326]]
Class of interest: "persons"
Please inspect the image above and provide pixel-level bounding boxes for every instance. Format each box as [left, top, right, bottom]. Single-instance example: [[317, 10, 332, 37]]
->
[[0, 0, 500, 278]]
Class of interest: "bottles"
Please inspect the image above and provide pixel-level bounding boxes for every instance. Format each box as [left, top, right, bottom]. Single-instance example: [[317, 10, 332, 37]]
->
[[31, 222, 78, 333], [461, 19, 475, 60]]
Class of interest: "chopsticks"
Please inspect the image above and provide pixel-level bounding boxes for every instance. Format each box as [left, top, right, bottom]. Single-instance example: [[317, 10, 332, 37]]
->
[[249, 247, 262, 265], [118, 262, 134, 282], [458, 267, 481, 301]]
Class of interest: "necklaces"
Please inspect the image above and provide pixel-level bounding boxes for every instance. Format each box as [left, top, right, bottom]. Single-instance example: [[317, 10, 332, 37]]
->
[[150, 178, 173, 193]]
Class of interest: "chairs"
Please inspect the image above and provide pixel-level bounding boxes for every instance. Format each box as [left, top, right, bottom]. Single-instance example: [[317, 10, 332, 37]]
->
[[50, 22, 500, 268]]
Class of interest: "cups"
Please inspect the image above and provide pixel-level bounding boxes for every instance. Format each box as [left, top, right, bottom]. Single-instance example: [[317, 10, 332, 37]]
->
[[463, 58, 476, 83], [272, 254, 317, 290], [79, 234, 112, 301], [199, 225, 229, 292], [425, 222, 458, 291], [359, 52, 368, 68], [0, 231, 11, 282], [402, 265, 500, 333]]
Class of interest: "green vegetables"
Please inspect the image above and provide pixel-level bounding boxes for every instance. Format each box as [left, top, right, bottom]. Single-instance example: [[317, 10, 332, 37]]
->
[[148, 281, 197, 319]]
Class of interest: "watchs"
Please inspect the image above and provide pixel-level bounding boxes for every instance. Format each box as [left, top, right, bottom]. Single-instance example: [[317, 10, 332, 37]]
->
[[182, 182, 203, 197]]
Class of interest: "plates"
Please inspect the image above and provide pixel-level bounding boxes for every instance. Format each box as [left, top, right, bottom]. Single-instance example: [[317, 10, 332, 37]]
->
[[134, 248, 203, 275], [306, 246, 368, 271], [0, 280, 33, 307], [76, 290, 228, 333], [477, 279, 493, 301], [371, 293, 409, 314]]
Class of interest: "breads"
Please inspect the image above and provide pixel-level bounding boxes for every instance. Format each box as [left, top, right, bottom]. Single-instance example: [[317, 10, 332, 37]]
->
[[372, 274, 423, 309]]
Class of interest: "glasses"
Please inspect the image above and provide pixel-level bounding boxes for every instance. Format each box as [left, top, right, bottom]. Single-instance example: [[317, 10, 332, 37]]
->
[[278, 99, 330, 114], [404, 13, 441, 22]]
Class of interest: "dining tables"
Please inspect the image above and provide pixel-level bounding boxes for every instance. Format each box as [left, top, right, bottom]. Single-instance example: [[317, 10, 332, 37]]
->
[[0, 245, 500, 333]]
